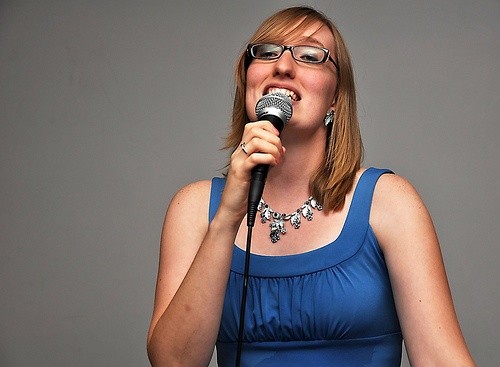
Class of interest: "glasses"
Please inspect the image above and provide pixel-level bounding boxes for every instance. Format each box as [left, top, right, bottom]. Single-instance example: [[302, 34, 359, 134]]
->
[[246, 40, 340, 73]]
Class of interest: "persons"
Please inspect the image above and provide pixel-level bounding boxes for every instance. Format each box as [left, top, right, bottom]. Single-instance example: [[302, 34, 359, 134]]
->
[[146, 6, 481, 367]]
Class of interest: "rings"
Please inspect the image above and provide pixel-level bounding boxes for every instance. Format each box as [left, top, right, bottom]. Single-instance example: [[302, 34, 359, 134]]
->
[[238, 143, 250, 156]]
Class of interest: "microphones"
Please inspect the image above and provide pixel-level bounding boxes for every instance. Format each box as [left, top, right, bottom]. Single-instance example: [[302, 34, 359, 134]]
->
[[247, 92, 294, 227]]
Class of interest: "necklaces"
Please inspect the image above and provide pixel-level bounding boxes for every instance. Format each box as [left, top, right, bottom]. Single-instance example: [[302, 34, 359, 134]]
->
[[257, 196, 326, 244]]
